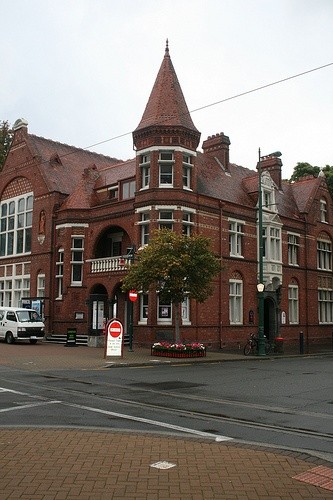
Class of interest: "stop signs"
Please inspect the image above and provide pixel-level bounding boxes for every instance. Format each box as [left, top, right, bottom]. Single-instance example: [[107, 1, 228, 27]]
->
[[106, 319, 124, 337], [129, 289, 138, 302]]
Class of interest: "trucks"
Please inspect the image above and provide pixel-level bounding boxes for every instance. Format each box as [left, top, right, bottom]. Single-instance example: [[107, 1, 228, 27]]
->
[[0, 306, 46, 344]]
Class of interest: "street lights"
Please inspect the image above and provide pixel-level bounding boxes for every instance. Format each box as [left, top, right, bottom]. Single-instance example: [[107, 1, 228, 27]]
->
[[256, 283, 268, 356]]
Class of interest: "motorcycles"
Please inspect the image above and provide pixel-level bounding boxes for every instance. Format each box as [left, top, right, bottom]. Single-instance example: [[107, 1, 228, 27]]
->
[[244, 332, 274, 355]]
